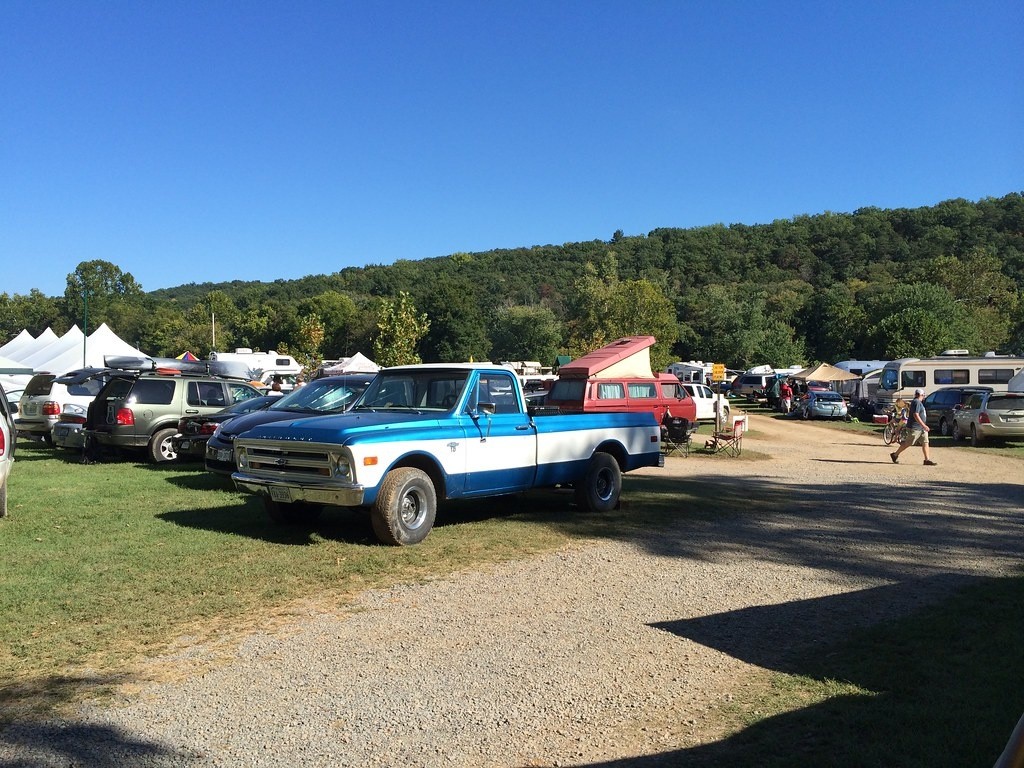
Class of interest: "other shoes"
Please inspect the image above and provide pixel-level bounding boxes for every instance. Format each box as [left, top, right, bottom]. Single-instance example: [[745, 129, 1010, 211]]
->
[[924, 460, 937, 465], [890, 452, 900, 464]]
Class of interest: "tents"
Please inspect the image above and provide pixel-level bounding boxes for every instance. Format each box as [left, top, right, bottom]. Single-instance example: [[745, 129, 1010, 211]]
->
[[322, 352, 385, 376], [0, 321, 153, 416], [788, 362, 862, 420]]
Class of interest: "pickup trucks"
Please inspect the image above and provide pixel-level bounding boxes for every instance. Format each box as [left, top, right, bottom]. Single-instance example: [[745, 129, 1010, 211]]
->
[[228, 362, 666, 547]]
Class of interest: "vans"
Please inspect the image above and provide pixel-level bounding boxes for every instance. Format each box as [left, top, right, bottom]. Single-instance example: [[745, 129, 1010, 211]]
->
[[544, 334, 699, 439]]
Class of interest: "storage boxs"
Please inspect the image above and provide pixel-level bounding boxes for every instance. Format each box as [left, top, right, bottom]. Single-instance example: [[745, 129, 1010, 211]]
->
[[873, 414, 888, 424]]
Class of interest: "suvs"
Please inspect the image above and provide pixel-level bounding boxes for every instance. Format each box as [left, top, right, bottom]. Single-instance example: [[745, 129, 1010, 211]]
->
[[204, 374, 498, 477], [922, 386, 996, 435], [1, 347, 310, 521], [952, 391, 1024, 447]]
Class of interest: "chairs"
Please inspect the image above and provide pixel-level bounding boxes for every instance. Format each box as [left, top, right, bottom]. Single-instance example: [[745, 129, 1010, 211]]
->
[[664, 416, 695, 459], [381, 382, 412, 407], [712, 419, 747, 459], [190, 391, 206, 405]]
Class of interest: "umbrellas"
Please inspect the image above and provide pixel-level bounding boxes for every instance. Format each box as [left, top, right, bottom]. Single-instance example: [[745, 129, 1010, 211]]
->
[[174, 351, 201, 361]]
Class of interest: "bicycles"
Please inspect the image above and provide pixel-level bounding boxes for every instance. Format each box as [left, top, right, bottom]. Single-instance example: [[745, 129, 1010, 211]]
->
[[882, 409, 911, 446]]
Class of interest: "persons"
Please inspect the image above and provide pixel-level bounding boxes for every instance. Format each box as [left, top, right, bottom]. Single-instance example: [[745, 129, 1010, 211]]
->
[[780, 382, 792, 415], [890, 389, 937, 466], [887, 397, 912, 444], [293, 374, 307, 390], [267, 382, 284, 396], [789, 380, 809, 396]]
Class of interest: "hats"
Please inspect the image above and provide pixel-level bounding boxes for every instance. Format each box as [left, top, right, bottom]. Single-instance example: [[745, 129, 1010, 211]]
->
[[915, 388, 925, 395]]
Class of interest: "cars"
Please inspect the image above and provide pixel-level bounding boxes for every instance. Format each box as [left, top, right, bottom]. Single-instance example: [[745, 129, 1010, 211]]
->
[[481, 372, 560, 407], [682, 383, 732, 425], [170, 394, 287, 456], [710, 373, 849, 423]]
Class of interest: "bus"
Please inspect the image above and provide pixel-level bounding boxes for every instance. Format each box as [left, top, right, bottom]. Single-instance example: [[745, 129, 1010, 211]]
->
[[874, 349, 1024, 409], [829, 358, 893, 396]]
